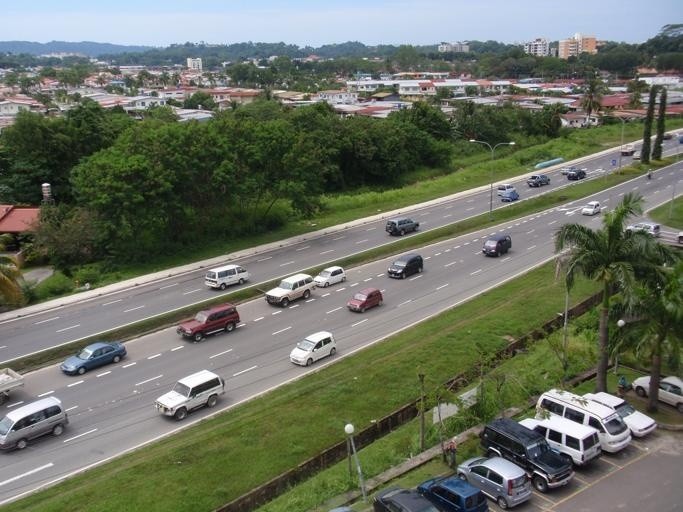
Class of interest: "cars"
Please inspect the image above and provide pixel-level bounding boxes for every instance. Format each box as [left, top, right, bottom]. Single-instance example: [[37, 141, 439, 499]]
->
[[347, 288, 383, 312], [561, 166, 575, 175], [679, 136, 683, 144], [60, 341, 127, 376], [631, 374, 683, 412], [632, 152, 640, 160], [288, 331, 335, 366], [663, 133, 671, 139], [581, 391, 658, 438], [314, 266, 347, 287], [677, 231, 683, 244], [372, 483, 442, 511]]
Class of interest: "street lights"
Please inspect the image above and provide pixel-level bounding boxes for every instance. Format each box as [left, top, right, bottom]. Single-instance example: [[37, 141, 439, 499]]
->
[[469, 139, 515, 212], [658, 143, 683, 221], [613, 320, 626, 375], [614, 112, 635, 170], [344, 423, 368, 503]]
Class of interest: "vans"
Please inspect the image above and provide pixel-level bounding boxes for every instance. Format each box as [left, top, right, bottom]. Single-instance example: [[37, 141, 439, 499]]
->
[[386, 253, 423, 280], [626, 223, 661, 237], [581, 201, 600, 216], [482, 235, 511, 256], [497, 184, 515, 196], [501, 190, 519, 202], [0, 395, 68, 450], [204, 264, 250, 291], [535, 388, 633, 455]]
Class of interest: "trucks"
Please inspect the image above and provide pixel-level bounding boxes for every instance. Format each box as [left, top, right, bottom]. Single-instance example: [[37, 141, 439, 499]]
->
[[0, 366, 24, 404], [619, 144, 635, 155]]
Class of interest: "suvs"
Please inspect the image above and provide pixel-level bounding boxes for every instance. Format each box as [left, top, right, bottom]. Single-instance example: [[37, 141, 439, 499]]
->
[[568, 169, 585, 180], [527, 175, 550, 187], [517, 417, 601, 469], [416, 475, 489, 511], [456, 456, 532, 509], [264, 273, 315, 307], [175, 303, 240, 342], [152, 369, 225, 419], [477, 417, 575, 492], [385, 217, 419, 236]]
